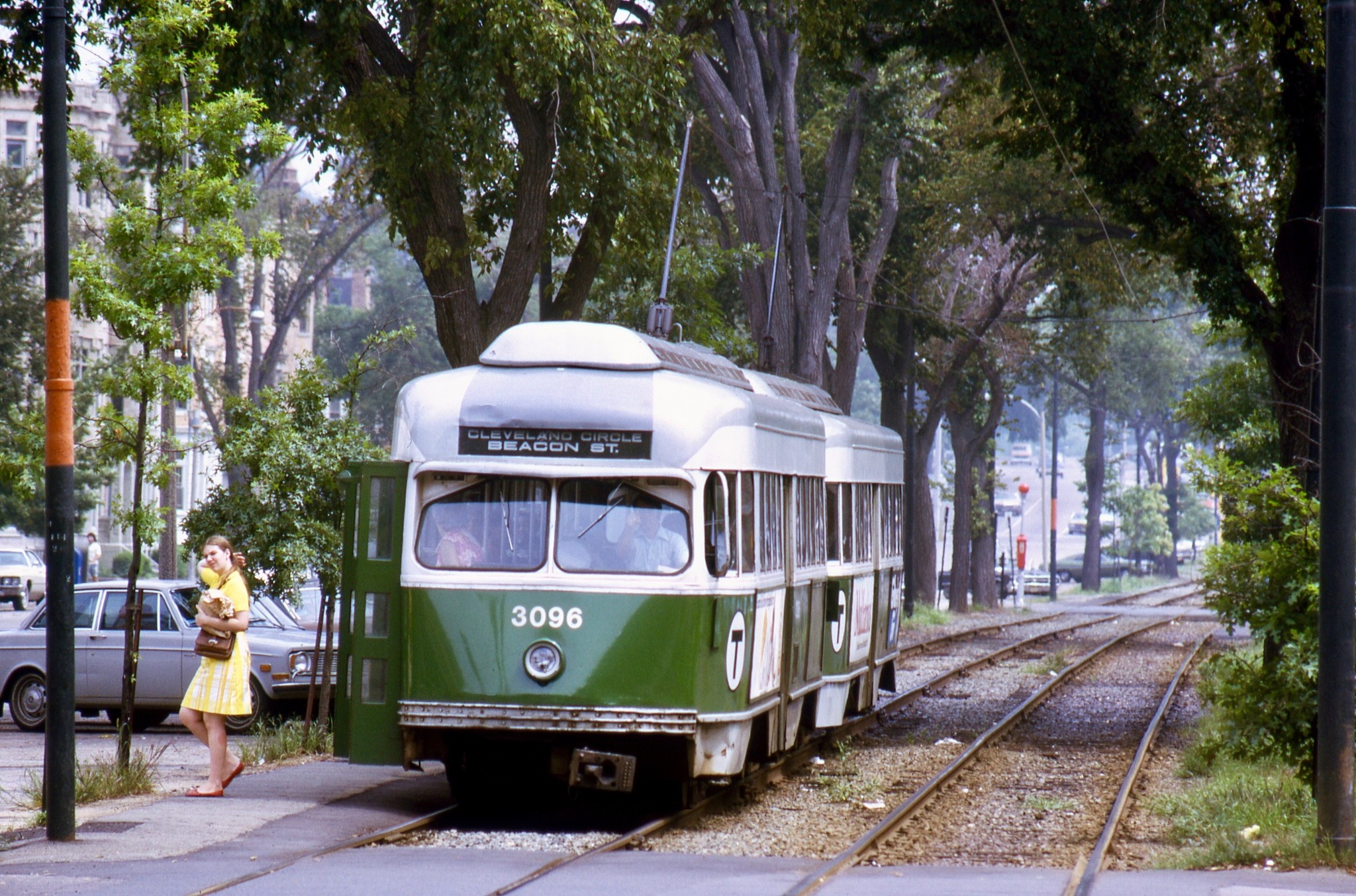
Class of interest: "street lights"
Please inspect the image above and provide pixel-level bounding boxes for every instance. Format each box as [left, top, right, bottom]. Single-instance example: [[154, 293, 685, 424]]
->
[[983, 391, 1049, 576], [184, 303, 266, 585]]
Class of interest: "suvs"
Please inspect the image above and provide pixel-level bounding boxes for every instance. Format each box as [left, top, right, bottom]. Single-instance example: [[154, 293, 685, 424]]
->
[[1009, 443, 1033, 465]]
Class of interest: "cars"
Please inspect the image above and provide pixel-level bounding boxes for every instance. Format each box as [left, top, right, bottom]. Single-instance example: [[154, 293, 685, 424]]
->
[[0, 580, 339, 736], [994, 492, 1022, 517], [1195, 492, 1220, 510], [939, 555, 1061, 600], [1051, 547, 1155, 584], [1068, 510, 1088, 534], [0, 548, 47, 610], [1037, 452, 1064, 478]]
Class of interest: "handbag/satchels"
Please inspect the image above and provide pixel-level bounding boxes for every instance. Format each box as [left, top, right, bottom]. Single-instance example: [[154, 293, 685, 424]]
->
[[193, 625, 236, 659]]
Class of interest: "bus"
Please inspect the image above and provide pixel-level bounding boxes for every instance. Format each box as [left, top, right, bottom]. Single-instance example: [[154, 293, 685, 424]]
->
[[332, 114, 906, 802]]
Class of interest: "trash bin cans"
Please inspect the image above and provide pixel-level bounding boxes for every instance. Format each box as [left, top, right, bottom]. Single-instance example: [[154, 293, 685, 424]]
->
[[73, 547, 83, 584]]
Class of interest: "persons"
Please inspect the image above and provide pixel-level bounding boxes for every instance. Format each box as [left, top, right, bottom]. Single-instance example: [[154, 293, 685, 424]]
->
[[178, 536, 251, 797], [617, 501, 688, 574], [507, 510, 540, 564], [87, 533, 101, 582], [435, 505, 485, 568]]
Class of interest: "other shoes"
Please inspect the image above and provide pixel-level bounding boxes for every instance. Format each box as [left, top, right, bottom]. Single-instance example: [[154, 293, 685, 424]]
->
[[221, 763, 246, 789], [185, 786, 224, 796]]
